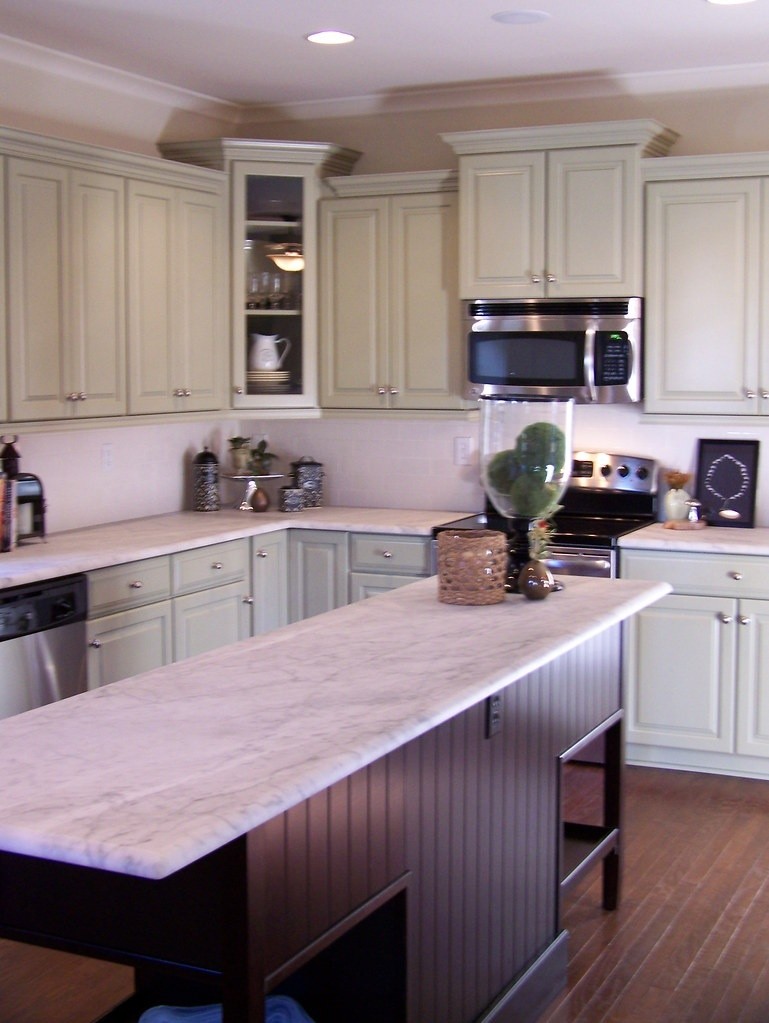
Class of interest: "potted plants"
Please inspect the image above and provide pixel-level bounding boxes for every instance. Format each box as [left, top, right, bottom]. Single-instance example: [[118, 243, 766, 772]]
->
[[227, 436, 278, 476]]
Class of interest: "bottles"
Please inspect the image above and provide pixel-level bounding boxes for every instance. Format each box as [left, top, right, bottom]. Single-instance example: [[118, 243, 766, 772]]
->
[[291, 455, 324, 509], [191, 446, 221, 512]]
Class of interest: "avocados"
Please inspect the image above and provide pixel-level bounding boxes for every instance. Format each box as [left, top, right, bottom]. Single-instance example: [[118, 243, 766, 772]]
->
[[489, 420, 567, 517]]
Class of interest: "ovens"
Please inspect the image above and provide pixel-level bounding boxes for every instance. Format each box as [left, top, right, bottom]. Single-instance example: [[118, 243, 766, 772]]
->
[[430, 540, 617, 579], [5, 472, 45, 545]]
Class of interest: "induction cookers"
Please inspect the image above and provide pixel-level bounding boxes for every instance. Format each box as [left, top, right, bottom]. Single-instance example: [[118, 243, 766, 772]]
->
[[432, 449, 658, 547]]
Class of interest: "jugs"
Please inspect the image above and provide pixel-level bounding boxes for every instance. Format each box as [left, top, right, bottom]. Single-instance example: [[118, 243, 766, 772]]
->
[[248, 334, 291, 371]]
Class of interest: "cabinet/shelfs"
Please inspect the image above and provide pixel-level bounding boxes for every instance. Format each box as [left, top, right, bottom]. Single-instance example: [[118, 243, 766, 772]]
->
[[87, 527, 432, 689], [1, 117, 768, 431], [614, 550, 767, 782]]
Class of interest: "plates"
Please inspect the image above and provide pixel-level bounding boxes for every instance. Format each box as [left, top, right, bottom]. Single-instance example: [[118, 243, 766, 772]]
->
[[246, 371, 292, 394]]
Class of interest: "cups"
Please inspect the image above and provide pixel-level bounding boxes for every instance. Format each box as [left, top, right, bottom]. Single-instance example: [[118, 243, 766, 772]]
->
[[279, 485, 303, 511], [247, 270, 301, 310]]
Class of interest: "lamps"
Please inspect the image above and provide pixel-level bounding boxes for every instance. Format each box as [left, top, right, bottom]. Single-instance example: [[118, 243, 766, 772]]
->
[[265, 251, 303, 273]]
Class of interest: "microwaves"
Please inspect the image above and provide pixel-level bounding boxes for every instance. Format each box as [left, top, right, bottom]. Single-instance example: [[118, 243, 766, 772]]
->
[[459, 297, 643, 403]]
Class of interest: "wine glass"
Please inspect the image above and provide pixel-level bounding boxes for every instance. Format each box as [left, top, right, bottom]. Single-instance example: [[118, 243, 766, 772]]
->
[[479, 393, 575, 592]]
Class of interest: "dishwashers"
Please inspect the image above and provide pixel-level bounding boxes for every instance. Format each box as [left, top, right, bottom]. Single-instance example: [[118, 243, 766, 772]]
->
[[0, 573, 88, 722]]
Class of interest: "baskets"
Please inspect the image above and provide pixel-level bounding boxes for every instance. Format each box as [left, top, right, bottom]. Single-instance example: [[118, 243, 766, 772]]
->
[[438, 531, 506, 606]]
